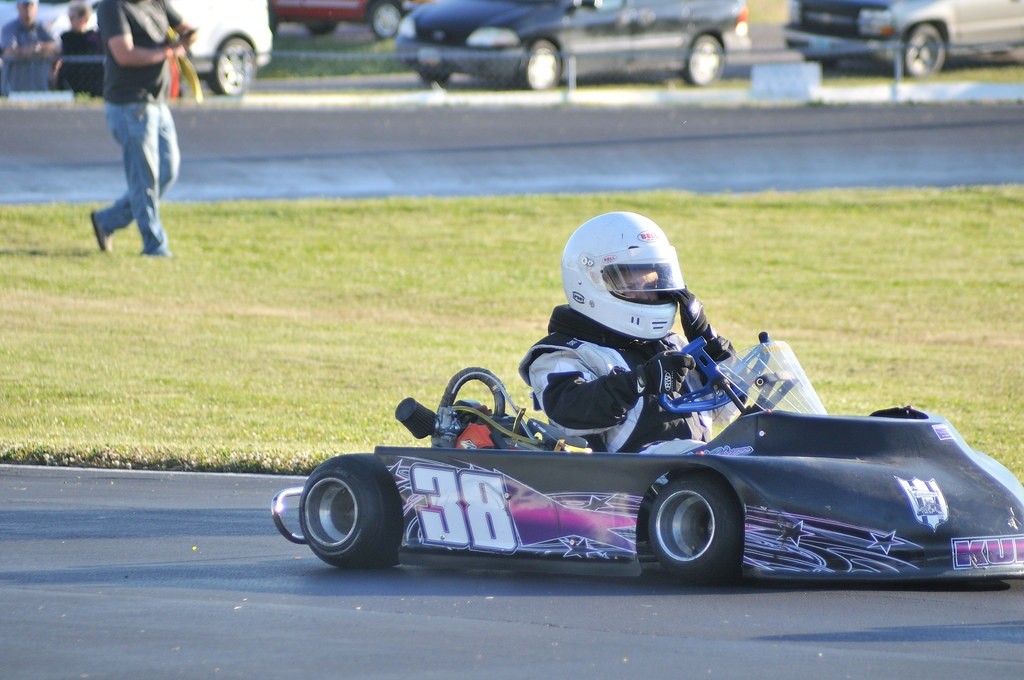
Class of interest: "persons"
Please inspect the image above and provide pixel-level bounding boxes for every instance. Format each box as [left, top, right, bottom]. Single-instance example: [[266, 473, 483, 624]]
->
[[90, 0, 197, 259], [0, 0, 106, 97], [520, 212, 749, 456]]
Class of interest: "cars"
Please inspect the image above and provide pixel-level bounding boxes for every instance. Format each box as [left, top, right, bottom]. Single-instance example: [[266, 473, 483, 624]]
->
[[781, 1, 1023, 83], [393, 0, 754, 93], [0, 0, 275, 97], [266, 0, 418, 39]]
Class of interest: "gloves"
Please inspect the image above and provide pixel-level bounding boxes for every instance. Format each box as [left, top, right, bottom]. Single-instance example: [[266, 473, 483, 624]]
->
[[672, 285, 729, 361], [631, 350, 696, 397]]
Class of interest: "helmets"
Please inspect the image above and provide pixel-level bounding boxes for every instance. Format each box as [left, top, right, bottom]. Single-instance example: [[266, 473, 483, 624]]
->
[[561, 212, 684, 341]]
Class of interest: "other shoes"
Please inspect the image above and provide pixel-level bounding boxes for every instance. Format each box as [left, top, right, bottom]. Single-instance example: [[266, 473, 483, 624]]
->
[[91, 211, 113, 252]]
